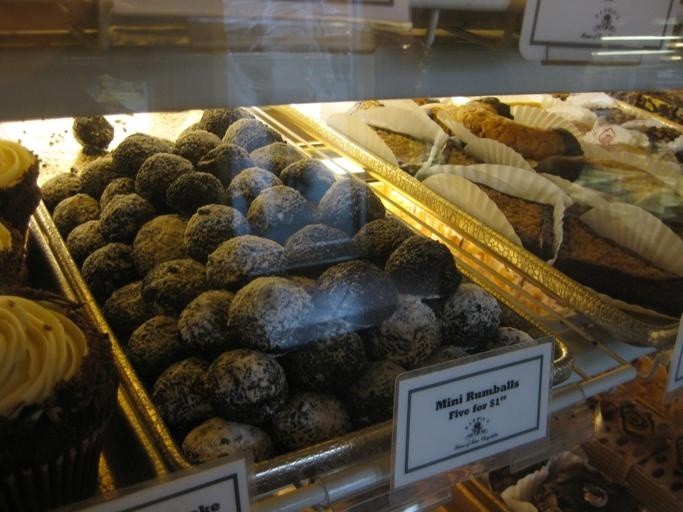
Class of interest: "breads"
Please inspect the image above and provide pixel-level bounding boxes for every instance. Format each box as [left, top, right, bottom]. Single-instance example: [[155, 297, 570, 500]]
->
[[327, 93, 681, 333], [42, 109, 540, 471]]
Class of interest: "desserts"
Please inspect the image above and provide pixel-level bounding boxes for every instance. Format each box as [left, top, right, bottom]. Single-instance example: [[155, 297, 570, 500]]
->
[[0, 137, 121, 508]]
